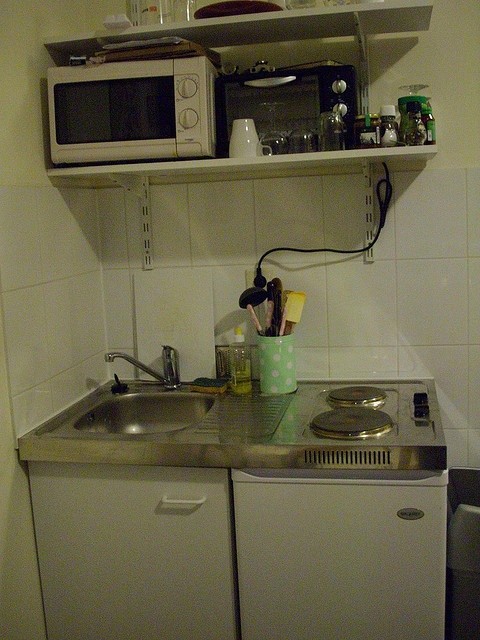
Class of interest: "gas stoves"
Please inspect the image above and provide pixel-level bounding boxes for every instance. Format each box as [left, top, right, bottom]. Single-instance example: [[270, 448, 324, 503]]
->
[[269, 378, 450, 471]]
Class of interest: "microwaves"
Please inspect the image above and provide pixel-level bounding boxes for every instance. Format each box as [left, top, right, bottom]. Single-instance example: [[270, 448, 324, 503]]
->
[[47, 54, 216, 163]]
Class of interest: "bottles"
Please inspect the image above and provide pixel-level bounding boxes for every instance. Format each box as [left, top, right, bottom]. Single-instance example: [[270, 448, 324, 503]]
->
[[315, 110, 345, 150], [405, 101, 425, 146], [381, 103, 399, 147], [420, 98, 437, 147], [357, 112, 381, 150]]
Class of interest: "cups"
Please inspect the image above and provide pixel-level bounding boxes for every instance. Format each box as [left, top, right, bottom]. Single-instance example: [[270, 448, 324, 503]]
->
[[321, 112, 345, 150], [256, 335, 298, 393], [228, 119, 262, 159]]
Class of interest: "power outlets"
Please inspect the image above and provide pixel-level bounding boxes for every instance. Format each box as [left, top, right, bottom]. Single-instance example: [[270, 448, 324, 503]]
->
[[245, 270, 273, 288]]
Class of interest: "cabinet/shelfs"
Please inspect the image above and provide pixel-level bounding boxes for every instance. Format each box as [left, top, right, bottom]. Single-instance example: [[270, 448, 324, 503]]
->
[[17, 440, 234, 636]]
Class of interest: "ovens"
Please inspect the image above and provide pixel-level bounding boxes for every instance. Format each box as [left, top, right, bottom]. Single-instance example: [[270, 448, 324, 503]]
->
[[214, 64, 358, 158]]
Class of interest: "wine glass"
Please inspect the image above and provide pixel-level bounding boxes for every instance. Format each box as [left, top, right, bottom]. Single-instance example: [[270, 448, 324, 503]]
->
[[259, 102, 286, 155], [286, 100, 320, 152]]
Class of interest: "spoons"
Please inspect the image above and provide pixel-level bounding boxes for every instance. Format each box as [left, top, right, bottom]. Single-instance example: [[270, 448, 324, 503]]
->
[[239, 284, 269, 336]]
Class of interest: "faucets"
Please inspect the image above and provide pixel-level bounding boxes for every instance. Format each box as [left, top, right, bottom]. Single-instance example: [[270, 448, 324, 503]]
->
[[104, 344, 182, 390]]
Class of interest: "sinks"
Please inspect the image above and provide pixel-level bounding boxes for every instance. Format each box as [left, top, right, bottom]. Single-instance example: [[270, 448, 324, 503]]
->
[[73, 392, 217, 443]]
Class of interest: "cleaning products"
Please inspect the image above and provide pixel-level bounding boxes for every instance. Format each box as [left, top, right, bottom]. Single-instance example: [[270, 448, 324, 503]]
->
[[228, 324, 252, 395]]
